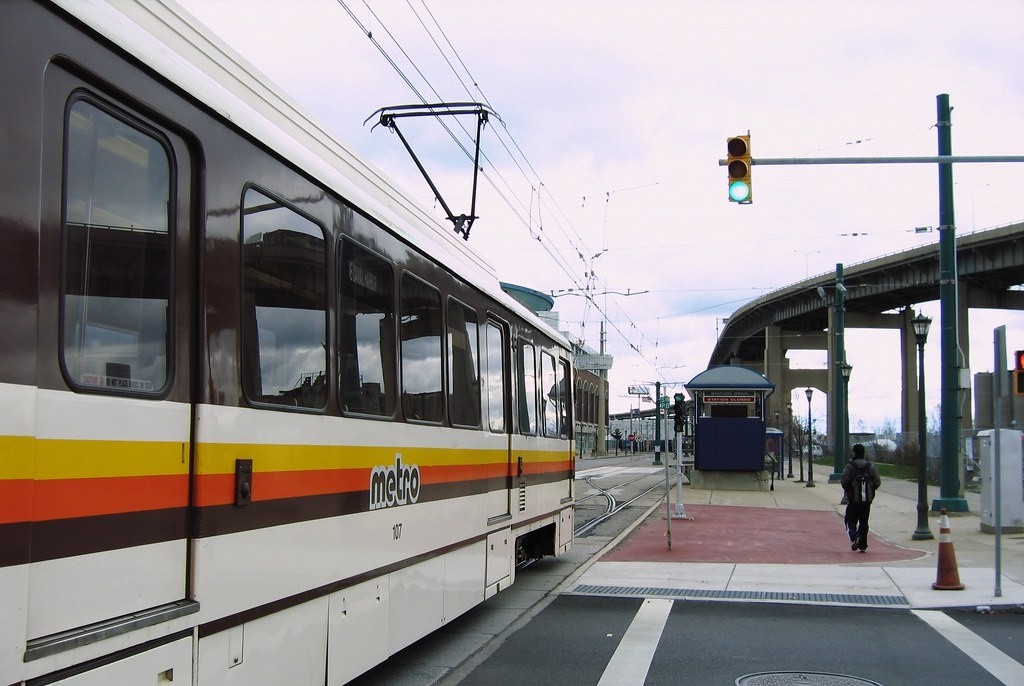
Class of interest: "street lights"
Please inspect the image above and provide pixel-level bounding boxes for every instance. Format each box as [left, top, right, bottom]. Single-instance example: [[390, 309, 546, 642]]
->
[[774, 410, 779, 429], [784, 402, 795, 478], [804, 388, 815, 487], [841, 362, 853, 468], [908, 311, 934, 541]]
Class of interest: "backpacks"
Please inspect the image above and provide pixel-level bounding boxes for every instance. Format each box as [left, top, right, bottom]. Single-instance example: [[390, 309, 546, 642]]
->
[[848, 460, 875, 507]]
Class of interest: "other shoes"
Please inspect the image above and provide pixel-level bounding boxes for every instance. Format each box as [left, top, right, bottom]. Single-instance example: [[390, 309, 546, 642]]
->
[[851, 534, 867, 553]]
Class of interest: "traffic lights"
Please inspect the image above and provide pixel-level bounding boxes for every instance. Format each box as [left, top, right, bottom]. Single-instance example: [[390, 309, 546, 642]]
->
[[1014, 350, 1024, 396], [725, 134, 755, 203], [673, 392, 683, 433]]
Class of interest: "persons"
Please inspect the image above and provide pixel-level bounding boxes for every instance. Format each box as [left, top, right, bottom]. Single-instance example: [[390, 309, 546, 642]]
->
[[839, 443, 883, 553]]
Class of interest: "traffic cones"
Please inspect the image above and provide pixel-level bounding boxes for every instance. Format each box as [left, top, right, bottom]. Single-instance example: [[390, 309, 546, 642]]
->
[[932, 506, 966, 590]]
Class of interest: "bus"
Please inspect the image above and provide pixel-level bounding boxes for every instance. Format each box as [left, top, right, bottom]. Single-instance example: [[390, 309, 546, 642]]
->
[[2, 0, 575, 686]]
